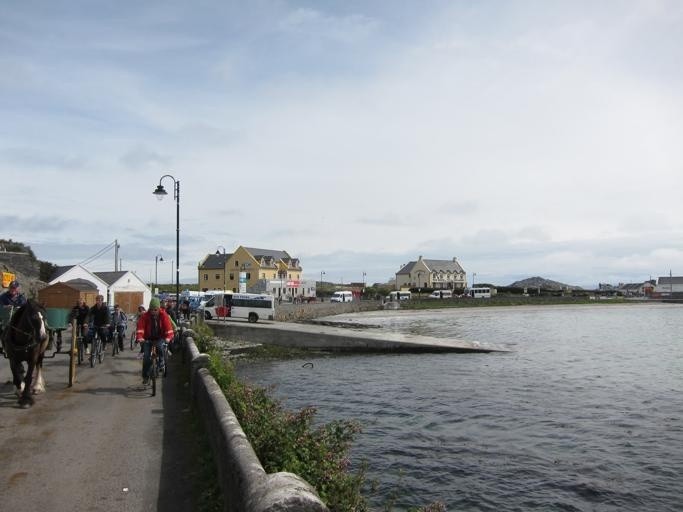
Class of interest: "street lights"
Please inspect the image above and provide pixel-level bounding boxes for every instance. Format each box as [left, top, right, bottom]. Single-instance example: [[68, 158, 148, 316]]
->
[[154, 173, 180, 320], [321, 270, 325, 302], [215, 245, 226, 323], [472, 272, 476, 288]]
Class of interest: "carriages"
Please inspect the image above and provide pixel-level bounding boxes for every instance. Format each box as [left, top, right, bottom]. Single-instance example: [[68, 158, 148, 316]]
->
[[0, 297, 78, 410]]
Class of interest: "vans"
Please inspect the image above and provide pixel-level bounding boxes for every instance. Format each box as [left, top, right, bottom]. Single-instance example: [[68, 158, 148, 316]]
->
[[386, 290, 411, 300], [330, 291, 352, 303], [429, 290, 452, 299]]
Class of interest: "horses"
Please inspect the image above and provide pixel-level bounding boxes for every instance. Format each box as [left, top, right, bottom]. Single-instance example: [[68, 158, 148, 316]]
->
[[0, 296, 50, 408]]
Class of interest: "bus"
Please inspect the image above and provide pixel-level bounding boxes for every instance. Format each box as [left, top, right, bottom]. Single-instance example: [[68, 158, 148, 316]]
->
[[464, 287, 491, 299], [179, 290, 274, 323]]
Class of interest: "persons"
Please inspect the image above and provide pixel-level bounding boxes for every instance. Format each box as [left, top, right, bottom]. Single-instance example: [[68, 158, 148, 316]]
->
[[131, 295, 192, 386], [0, 279, 28, 316], [67, 295, 128, 355]]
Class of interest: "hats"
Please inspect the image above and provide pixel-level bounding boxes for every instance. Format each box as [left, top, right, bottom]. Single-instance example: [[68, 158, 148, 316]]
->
[[149, 298, 160, 309], [9, 281, 19, 288]]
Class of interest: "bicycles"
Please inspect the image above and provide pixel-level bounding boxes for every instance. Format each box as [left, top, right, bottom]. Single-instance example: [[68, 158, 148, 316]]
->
[[77, 318, 180, 397]]
